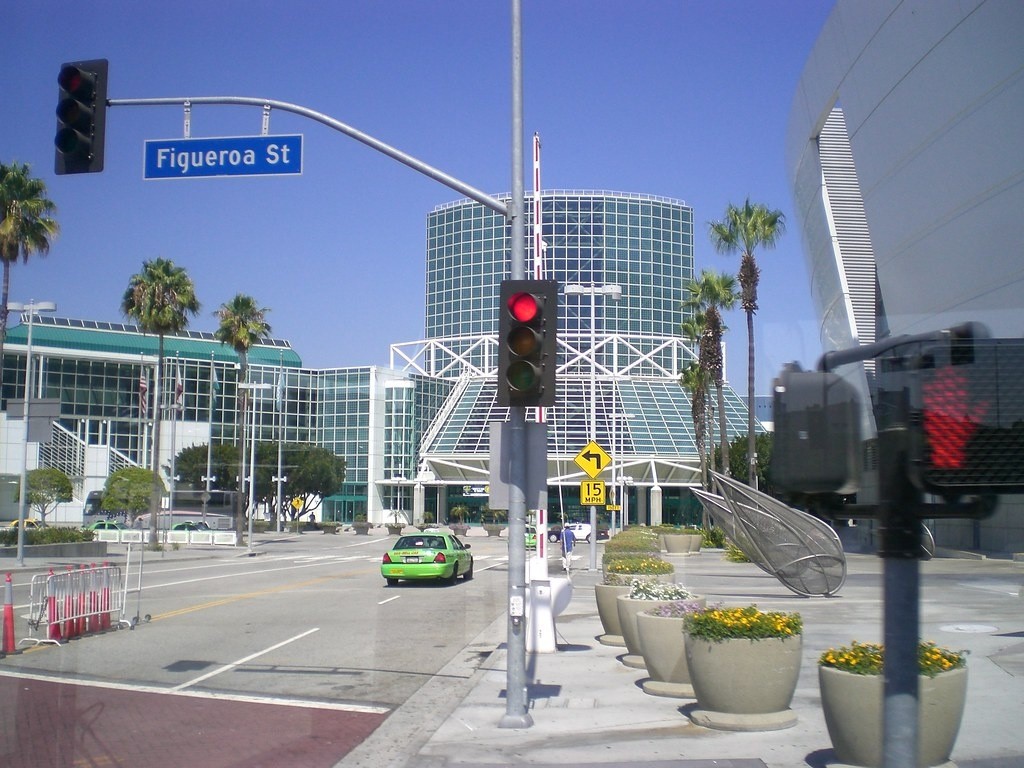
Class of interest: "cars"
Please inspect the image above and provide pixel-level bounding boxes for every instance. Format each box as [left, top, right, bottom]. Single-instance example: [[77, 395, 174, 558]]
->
[[172, 523, 207, 531], [381, 531, 476, 584], [10, 517, 50, 529], [507, 523, 536, 550], [88, 520, 130, 530]]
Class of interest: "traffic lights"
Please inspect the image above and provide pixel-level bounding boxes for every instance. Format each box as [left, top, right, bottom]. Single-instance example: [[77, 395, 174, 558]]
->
[[909, 337, 1023, 497], [507, 291, 552, 405], [54, 65, 98, 160]]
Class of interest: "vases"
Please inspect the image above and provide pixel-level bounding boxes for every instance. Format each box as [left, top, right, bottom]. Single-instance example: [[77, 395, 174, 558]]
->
[[689, 535, 702, 555], [819, 665, 967, 768], [604, 573, 675, 586], [686, 641, 803, 732], [664, 535, 691, 556], [658, 535, 667, 551], [635, 612, 696, 699], [595, 583, 627, 647], [455, 530, 467, 537], [616, 594, 707, 669]]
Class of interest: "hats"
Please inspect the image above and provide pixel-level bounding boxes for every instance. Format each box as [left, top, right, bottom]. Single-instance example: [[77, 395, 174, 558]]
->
[[564, 523, 570, 528]]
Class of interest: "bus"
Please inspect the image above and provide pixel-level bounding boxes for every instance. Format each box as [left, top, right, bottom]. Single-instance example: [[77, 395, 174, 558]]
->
[[83, 489, 244, 532]]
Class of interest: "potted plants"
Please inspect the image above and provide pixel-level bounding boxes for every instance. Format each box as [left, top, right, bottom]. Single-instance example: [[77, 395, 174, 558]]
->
[[287, 522, 306, 533], [353, 521, 372, 535], [417, 512, 435, 531], [384, 511, 404, 535], [253, 521, 270, 532], [483, 505, 506, 537], [318, 521, 340, 534]]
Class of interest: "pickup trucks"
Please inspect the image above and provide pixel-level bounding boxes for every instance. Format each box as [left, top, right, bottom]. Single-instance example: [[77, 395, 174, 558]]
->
[[548, 523, 610, 543]]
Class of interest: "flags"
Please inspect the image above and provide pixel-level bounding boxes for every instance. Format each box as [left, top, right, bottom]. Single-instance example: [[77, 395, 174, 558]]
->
[[276, 357, 285, 411], [139, 355, 147, 416], [209, 356, 220, 411], [174, 354, 183, 411]]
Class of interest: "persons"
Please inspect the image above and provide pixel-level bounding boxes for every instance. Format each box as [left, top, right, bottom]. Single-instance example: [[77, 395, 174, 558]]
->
[[407, 538, 438, 547], [309, 512, 315, 522], [560, 523, 575, 571]]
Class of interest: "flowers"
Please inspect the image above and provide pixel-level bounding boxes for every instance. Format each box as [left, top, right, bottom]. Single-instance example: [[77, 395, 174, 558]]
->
[[448, 505, 470, 529], [645, 601, 718, 618], [817, 638, 971, 678], [628, 579, 692, 600], [607, 557, 674, 574], [682, 605, 801, 641], [600, 573, 637, 586]]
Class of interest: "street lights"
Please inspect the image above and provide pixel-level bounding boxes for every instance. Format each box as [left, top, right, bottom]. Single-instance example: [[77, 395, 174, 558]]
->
[[5, 299, 59, 567], [533, 282, 624, 653]]
